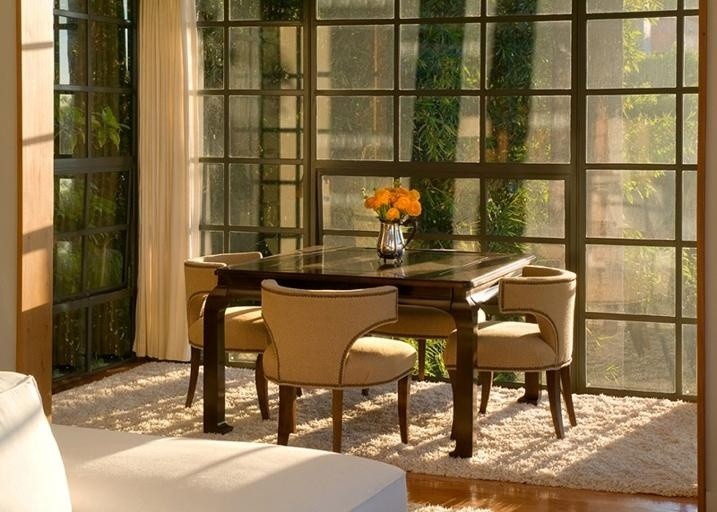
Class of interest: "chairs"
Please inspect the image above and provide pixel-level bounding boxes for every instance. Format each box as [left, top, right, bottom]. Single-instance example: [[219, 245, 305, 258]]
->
[[443, 267, 577, 440], [258, 278, 415, 455], [184, 249, 300, 420]]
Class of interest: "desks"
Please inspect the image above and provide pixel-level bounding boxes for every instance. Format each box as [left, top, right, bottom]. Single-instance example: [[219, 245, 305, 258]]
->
[[205, 244, 536, 460]]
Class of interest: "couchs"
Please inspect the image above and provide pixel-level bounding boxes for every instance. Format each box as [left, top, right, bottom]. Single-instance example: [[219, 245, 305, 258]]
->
[[1, 369, 405, 512]]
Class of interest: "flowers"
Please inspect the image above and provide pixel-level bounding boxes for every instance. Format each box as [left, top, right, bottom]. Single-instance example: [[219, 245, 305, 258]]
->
[[365, 188, 422, 222]]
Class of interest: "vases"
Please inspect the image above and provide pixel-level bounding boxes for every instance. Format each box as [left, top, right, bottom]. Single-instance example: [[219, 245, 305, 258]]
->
[[379, 221, 413, 261]]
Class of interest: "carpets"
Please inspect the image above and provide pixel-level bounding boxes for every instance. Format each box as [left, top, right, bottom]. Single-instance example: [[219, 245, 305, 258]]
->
[[404, 493, 492, 512], [48, 358, 698, 496]]
